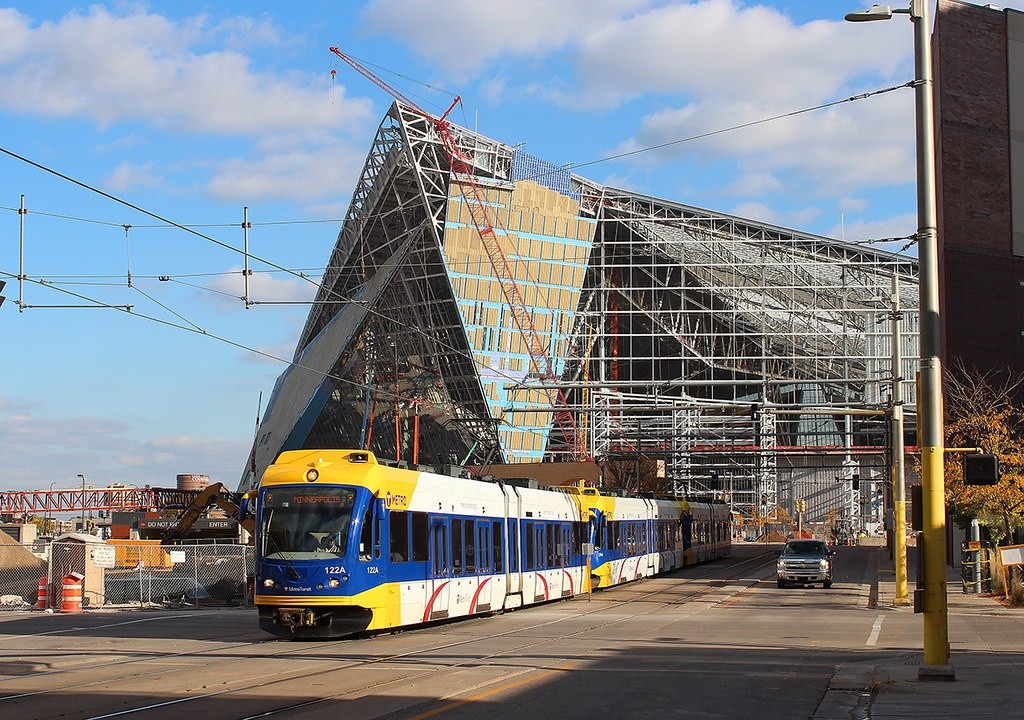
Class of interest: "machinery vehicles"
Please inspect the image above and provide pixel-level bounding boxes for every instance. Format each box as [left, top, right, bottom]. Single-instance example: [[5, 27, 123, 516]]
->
[[834, 519, 852, 544], [160, 482, 255, 608]]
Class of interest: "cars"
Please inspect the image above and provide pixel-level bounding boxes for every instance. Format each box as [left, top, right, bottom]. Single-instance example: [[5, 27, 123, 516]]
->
[[747, 537, 755, 542], [876, 531, 884, 538]]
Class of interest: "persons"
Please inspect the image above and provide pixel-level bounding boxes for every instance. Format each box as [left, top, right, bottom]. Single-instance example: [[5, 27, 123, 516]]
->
[[312, 533, 345, 555]]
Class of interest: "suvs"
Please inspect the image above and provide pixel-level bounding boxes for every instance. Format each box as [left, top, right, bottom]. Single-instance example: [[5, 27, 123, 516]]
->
[[774, 539, 837, 589]]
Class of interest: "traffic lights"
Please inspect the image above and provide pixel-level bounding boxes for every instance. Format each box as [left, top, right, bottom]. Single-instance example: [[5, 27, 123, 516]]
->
[[795, 500, 800, 513], [800, 501, 806, 511]]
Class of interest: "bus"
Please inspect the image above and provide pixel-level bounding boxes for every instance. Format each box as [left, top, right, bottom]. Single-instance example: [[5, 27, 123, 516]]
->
[[240, 449, 732, 643]]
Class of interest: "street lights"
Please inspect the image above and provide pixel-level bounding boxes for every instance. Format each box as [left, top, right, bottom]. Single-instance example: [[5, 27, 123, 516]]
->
[[77, 474, 85, 531], [49, 482, 57, 530], [844, 5, 958, 681], [860, 297, 911, 607]]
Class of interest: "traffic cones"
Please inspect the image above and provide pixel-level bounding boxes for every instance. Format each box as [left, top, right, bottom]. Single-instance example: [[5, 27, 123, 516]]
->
[[846, 538, 850, 547], [829, 540, 833, 546]]
